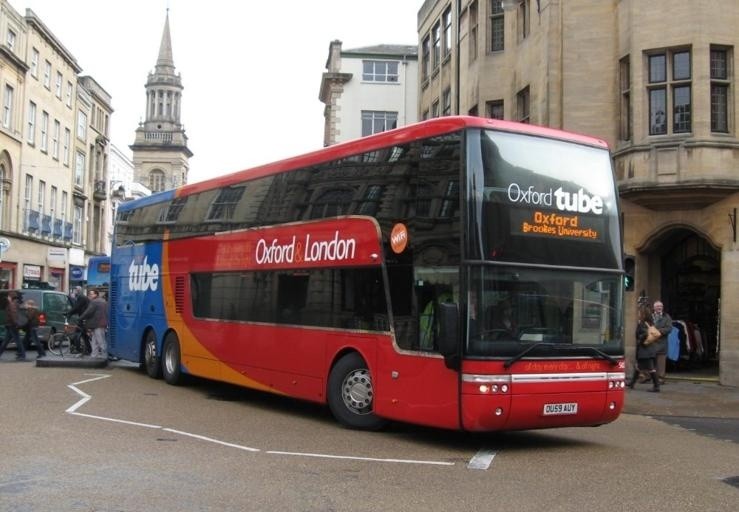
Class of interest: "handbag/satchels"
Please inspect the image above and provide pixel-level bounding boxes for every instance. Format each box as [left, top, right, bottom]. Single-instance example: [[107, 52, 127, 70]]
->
[[642, 320, 661, 344]]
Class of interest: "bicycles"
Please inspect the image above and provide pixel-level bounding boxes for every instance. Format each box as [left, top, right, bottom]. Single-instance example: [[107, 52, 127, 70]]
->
[[48, 314, 122, 361]]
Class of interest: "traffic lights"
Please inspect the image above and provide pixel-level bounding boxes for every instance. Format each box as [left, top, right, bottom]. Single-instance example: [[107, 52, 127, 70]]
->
[[622, 253, 636, 292]]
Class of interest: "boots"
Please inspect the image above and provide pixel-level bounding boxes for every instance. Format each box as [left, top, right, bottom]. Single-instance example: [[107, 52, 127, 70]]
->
[[647, 371, 660, 392], [626, 370, 640, 390]]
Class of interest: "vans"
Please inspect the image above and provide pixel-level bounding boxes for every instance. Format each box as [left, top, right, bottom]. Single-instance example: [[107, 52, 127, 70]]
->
[[0, 288, 74, 348]]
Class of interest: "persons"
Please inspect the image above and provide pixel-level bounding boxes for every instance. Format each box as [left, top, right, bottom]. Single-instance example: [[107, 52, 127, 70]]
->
[[624, 305, 660, 392], [0, 290, 25, 360], [62, 286, 108, 360], [18, 300, 46, 358], [651, 301, 672, 384]]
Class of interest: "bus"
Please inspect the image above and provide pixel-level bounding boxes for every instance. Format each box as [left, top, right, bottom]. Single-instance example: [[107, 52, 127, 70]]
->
[[87, 255, 111, 301], [106, 114, 626, 432]]
